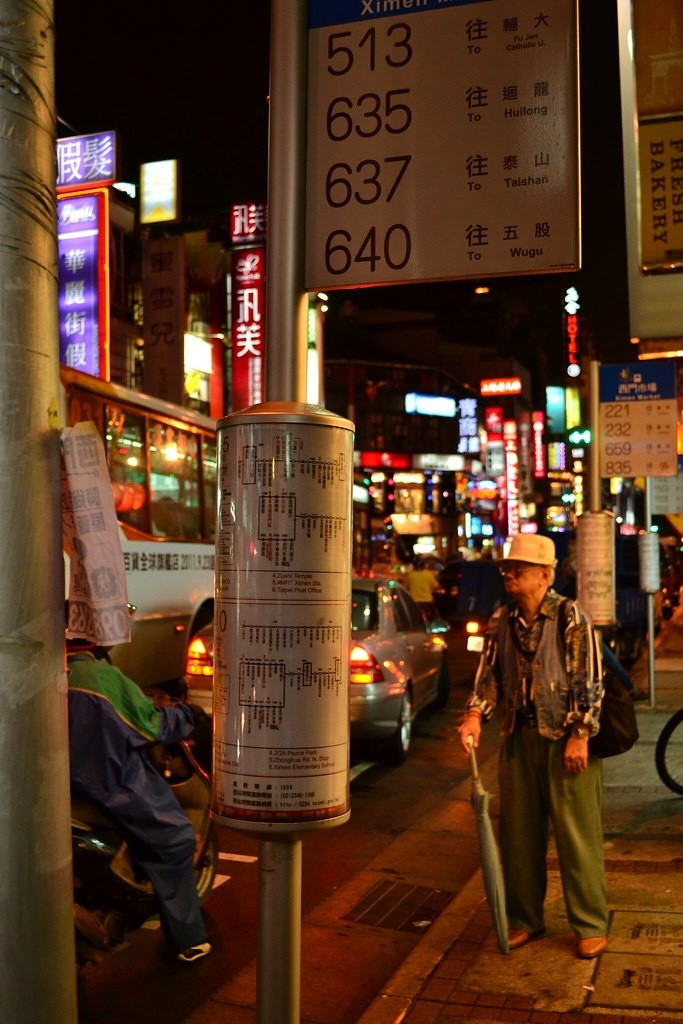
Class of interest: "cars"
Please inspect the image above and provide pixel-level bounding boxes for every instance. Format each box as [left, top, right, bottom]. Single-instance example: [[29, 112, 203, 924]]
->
[[183, 576, 452, 759], [452, 595, 510, 686]]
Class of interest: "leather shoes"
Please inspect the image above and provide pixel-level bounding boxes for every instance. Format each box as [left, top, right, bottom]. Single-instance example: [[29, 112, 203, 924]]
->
[[497, 928, 546, 950], [576, 936, 607, 959]]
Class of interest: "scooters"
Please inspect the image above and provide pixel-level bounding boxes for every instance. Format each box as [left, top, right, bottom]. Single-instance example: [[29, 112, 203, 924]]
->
[[69, 677, 221, 978]]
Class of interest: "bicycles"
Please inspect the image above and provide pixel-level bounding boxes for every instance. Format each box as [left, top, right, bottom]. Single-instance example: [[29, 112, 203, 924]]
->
[[654, 707, 683, 797]]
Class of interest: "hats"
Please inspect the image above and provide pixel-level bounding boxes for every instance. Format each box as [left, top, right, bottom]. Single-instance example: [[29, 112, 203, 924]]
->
[[494, 533, 558, 566]]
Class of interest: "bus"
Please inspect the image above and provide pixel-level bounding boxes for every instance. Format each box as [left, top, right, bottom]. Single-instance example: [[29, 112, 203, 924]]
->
[[53, 359, 221, 684]]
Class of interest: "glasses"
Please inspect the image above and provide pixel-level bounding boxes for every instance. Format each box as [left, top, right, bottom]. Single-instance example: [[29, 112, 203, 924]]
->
[[500, 565, 544, 577]]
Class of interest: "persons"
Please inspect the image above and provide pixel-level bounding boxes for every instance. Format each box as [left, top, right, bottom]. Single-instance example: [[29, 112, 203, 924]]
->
[[457, 532, 608, 959], [66, 658, 217, 975], [403, 555, 440, 622]]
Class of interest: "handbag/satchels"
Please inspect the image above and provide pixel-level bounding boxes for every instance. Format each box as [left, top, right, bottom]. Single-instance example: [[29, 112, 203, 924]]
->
[[558, 599, 640, 760]]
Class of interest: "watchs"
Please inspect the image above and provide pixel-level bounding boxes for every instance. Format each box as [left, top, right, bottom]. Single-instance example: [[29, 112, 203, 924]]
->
[[569, 728, 589, 739]]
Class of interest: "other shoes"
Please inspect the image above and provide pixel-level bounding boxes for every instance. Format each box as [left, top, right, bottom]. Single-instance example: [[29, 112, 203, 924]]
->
[[176, 938, 215, 961]]
